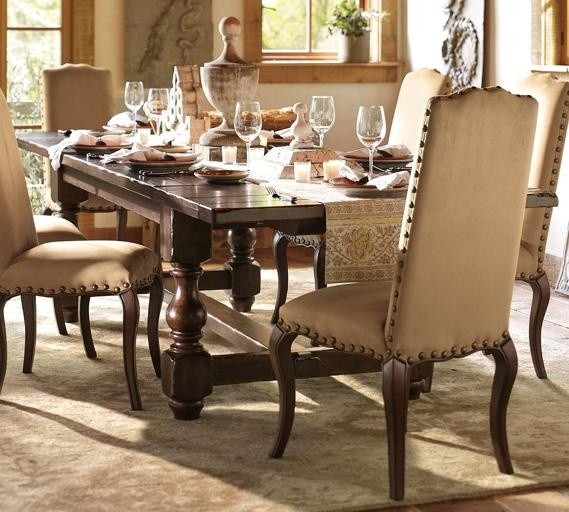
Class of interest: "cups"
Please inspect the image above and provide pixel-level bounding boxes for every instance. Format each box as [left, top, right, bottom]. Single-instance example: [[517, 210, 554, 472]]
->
[[294, 157, 311, 183], [221, 146, 238, 166], [323, 160, 344, 181], [250, 148, 264, 166]]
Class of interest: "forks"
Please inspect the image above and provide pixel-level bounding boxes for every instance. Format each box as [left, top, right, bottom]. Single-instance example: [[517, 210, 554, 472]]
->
[[264, 184, 296, 203], [137, 164, 205, 177]]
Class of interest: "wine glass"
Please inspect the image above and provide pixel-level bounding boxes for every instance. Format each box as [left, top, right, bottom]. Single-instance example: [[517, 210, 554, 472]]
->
[[309, 95, 335, 146], [234, 102, 262, 167], [356, 104, 387, 185], [124, 80, 145, 141], [147, 89, 170, 138]]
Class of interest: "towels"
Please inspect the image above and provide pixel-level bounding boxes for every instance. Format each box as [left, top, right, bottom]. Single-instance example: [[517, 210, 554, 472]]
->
[[367, 171, 410, 191], [48, 132, 122, 170], [343, 144, 414, 159], [100, 145, 202, 163], [189, 161, 250, 172]]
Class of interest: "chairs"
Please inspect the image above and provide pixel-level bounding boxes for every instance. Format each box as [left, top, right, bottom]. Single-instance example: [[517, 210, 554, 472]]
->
[[0, 89, 163, 410], [268, 86, 538, 500], [274, 70, 451, 323], [43, 64, 127, 240], [32, 216, 97, 358], [516, 74, 569, 379]]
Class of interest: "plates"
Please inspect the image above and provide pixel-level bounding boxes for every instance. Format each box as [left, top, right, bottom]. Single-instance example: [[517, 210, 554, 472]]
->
[[72, 140, 131, 155], [340, 155, 412, 165], [150, 145, 191, 153], [194, 171, 250, 183], [330, 176, 408, 189], [77, 130, 125, 136], [118, 156, 203, 170]]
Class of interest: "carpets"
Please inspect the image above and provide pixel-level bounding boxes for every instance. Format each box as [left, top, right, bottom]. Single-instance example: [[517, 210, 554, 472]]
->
[[0, 280, 569, 512]]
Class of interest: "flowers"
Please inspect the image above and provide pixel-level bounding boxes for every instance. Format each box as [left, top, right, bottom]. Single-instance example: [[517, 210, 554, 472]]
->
[[328, 0, 389, 63]]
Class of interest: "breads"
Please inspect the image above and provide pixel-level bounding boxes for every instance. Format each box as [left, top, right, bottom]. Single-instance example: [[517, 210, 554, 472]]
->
[[148, 101, 166, 115], [202, 107, 300, 131]]
